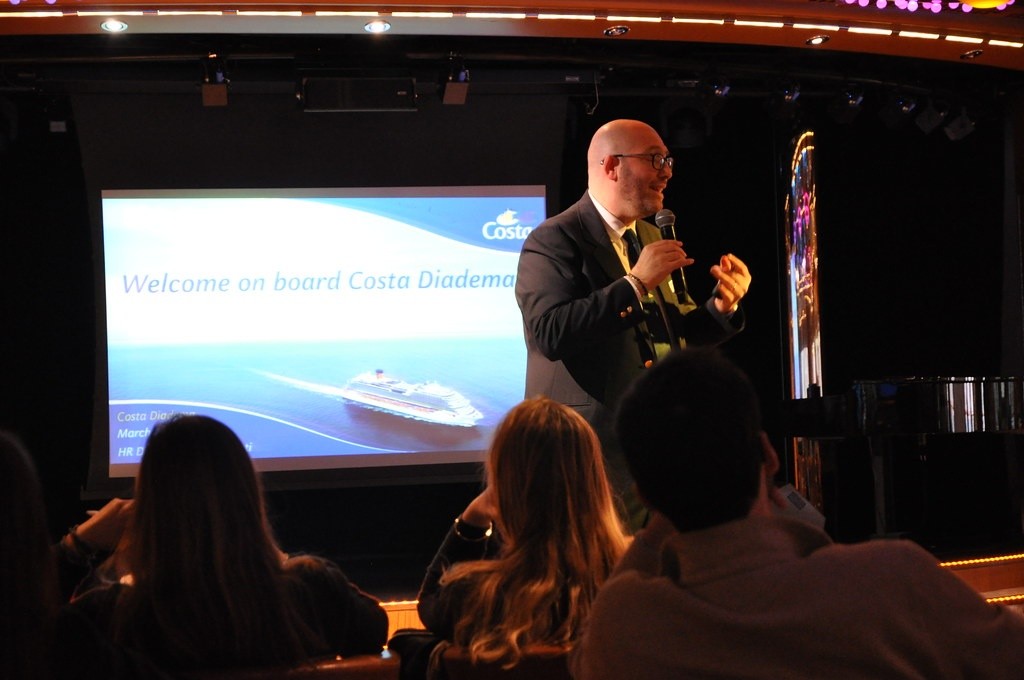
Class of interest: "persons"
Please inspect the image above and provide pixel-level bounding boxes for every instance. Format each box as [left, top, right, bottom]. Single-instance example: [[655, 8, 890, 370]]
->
[[568, 346, 1023, 680], [0, 413, 389, 680], [514, 119, 752, 510], [405, 398, 623, 678]]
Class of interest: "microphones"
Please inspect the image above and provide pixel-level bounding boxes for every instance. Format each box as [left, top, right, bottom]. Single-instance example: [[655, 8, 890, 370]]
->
[[655, 209, 686, 304]]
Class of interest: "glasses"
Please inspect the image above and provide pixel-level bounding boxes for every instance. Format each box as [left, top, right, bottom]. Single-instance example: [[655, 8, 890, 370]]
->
[[600, 153, 674, 172]]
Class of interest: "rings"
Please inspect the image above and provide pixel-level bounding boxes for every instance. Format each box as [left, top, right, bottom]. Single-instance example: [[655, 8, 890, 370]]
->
[[731, 283, 737, 291]]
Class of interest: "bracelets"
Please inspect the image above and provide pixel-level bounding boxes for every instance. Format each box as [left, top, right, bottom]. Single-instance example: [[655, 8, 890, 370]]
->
[[627, 273, 648, 296], [455, 517, 492, 542], [70, 524, 113, 568]]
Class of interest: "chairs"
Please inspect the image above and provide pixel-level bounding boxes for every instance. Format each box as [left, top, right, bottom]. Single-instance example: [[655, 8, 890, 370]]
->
[[231, 648, 401, 680], [440, 639, 569, 680]]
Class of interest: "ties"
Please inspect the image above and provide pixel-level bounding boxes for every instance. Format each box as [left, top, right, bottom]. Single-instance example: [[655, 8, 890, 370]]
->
[[624, 229, 671, 361]]
